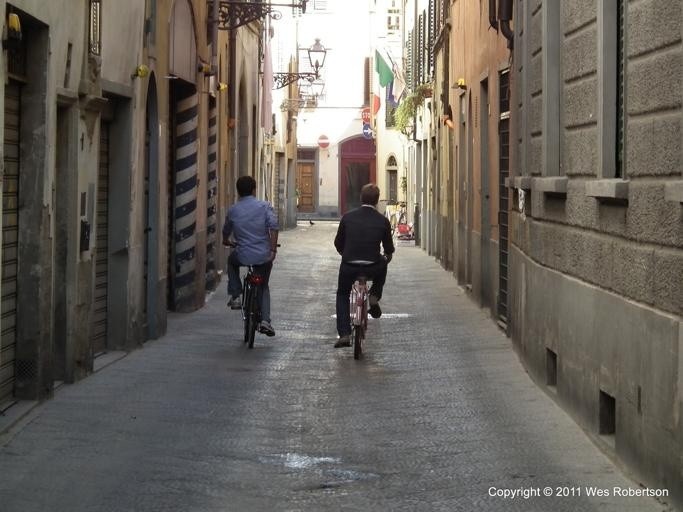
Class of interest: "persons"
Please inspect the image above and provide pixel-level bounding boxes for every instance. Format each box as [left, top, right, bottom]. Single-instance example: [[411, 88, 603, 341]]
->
[[334, 183, 395, 348], [222, 176, 279, 337]]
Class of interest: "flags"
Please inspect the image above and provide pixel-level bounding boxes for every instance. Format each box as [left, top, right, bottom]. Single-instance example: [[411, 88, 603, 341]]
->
[[374, 50, 393, 88], [388, 64, 406, 107]]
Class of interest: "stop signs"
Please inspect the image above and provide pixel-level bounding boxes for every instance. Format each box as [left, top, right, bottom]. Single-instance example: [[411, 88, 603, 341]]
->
[[318, 135, 329, 147], [361, 107, 370, 125]]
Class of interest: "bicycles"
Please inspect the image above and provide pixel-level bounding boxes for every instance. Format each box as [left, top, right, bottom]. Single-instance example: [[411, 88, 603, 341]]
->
[[339, 274, 379, 358], [226, 242, 281, 347], [380, 198, 407, 236]]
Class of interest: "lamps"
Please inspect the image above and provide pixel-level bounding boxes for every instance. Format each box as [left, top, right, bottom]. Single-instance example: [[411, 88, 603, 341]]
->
[[273, 38, 333, 90]]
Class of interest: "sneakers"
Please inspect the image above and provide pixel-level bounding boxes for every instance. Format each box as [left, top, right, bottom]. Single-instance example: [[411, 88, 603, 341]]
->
[[260, 320, 275, 336], [334, 336, 350, 348], [369, 302, 382, 318], [227, 297, 241, 307]]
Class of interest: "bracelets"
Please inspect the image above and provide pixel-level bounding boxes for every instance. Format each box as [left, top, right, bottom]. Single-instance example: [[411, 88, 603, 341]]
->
[[271, 249, 276, 254]]
